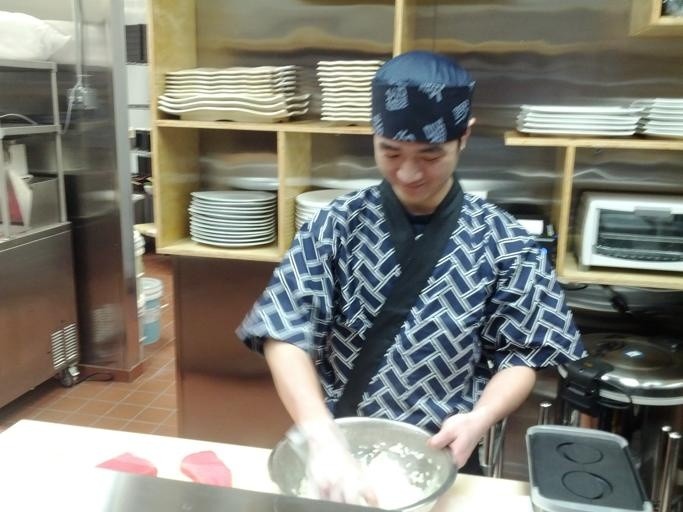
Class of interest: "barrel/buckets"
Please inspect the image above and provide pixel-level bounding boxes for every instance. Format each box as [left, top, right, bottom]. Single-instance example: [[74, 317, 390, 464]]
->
[[134, 231, 164, 345]]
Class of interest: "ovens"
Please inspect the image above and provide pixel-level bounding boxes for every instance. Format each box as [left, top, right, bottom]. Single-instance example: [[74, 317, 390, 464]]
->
[[575, 192, 683, 272]]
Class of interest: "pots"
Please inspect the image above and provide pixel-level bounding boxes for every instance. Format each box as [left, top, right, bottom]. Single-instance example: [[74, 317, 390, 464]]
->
[[555, 332, 682, 512]]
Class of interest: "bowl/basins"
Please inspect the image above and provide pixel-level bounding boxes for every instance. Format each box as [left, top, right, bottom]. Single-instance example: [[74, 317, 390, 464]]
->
[[267, 416, 458, 512]]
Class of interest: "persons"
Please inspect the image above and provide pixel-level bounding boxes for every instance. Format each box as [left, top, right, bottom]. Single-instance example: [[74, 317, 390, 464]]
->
[[232, 50, 592, 511]]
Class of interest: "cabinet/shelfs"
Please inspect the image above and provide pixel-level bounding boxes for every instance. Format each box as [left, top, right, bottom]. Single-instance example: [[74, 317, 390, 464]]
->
[[1, 60, 82, 406], [146, 0, 683, 290]]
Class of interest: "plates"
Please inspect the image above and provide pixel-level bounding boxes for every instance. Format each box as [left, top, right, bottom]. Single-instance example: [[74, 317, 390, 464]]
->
[[515, 96, 683, 139], [188, 190, 356, 247], [157, 59, 384, 125]]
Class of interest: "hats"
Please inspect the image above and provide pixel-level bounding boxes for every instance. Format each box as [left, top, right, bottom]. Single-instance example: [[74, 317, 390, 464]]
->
[[367, 50, 477, 143]]
